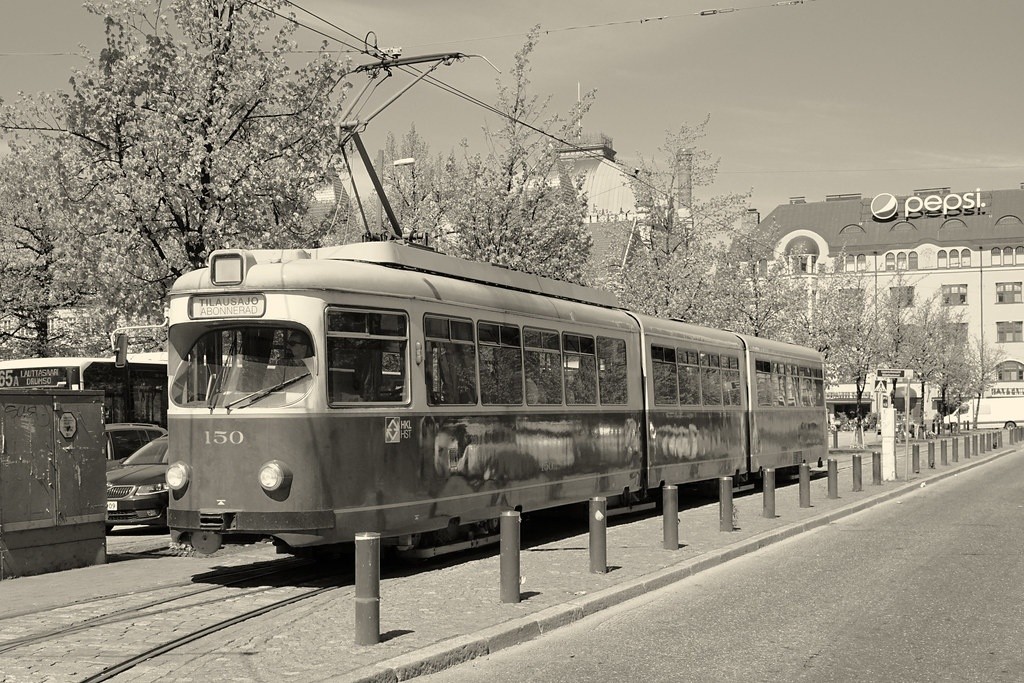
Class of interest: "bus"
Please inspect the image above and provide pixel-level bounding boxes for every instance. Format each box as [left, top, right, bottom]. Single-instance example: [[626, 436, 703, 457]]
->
[[0, 352, 244, 423], [110, 54, 828, 562]]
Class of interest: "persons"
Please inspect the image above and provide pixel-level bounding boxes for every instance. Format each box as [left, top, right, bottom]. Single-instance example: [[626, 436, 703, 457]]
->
[[273, 331, 310, 394], [570, 357, 615, 404]]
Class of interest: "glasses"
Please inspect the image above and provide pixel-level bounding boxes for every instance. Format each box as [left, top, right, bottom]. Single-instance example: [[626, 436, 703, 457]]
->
[[285, 339, 303, 346]]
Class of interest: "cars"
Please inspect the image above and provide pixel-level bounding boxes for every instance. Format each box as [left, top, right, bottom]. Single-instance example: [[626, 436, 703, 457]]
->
[[876, 413, 905, 435]]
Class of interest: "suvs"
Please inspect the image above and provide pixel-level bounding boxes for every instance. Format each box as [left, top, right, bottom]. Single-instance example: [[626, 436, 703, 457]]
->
[[105, 421, 167, 473], [102, 431, 168, 536]]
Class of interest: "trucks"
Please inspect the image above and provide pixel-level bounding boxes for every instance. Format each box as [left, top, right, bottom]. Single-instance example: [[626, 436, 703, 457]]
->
[[938, 397, 1024, 430]]
